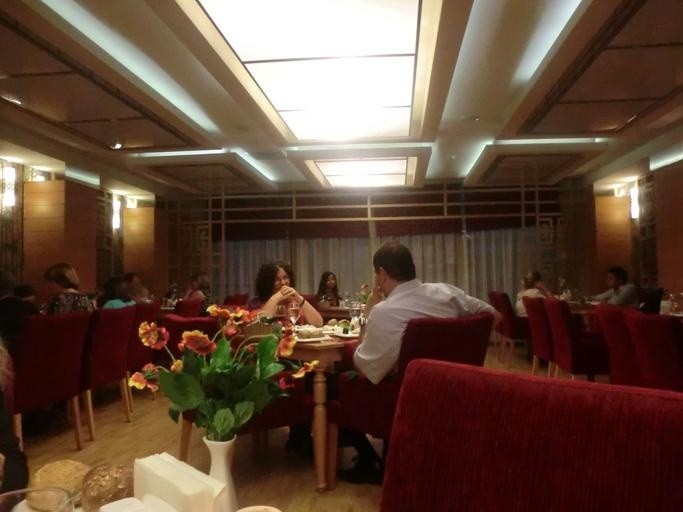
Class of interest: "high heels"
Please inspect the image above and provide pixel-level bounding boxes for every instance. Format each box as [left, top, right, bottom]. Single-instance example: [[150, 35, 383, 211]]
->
[[285, 440, 312, 460]]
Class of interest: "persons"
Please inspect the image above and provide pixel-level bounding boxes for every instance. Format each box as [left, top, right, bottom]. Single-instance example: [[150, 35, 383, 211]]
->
[[1, 340, 29, 491], [246, 262, 323, 460], [184, 272, 216, 316], [102, 278, 137, 309], [0, 271, 38, 344], [311, 271, 344, 308], [586, 266, 641, 307], [337, 243, 503, 484], [124, 272, 151, 303], [44, 262, 91, 312], [514, 270, 553, 336]]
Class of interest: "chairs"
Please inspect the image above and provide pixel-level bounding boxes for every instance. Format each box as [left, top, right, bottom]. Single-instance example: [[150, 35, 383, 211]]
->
[[11, 310, 85, 452], [326, 312, 496, 491], [543, 297, 607, 381], [298, 294, 316, 322], [498, 290, 531, 370], [376, 356, 681, 511], [594, 302, 641, 385], [80, 304, 134, 442], [230, 295, 246, 305], [223, 295, 232, 306], [521, 295, 554, 376], [127, 301, 162, 413], [175, 297, 202, 318], [488, 290, 498, 311]]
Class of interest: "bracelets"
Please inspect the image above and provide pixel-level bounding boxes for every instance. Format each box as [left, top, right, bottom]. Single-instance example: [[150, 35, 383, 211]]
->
[[358, 318, 371, 326], [298, 297, 306, 307]]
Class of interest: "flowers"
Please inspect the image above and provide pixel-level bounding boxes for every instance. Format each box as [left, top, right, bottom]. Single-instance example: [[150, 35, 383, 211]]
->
[[128, 322, 318, 439]]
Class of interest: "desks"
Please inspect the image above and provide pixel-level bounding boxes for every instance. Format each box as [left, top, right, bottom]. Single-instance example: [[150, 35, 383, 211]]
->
[[246, 324, 359, 491]]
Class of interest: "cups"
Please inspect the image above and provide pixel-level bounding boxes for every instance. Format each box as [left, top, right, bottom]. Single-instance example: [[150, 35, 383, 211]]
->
[[0, 486, 71, 511]]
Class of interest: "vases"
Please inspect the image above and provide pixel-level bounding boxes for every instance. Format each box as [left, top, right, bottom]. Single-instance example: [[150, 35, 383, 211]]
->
[[202, 433, 235, 512]]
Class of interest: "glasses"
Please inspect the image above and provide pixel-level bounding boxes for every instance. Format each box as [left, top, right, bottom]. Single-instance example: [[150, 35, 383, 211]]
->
[[273, 275, 291, 286]]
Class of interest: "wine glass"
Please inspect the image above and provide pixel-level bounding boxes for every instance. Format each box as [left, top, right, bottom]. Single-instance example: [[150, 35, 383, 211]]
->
[[289, 303, 301, 336], [344, 291, 350, 309], [350, 302, 361, 328], [337, 292, 343, 309]]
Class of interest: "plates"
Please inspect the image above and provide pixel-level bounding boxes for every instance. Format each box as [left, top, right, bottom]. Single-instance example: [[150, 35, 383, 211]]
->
[[292, 331, 333, 342], [334, 329, 359, 339]]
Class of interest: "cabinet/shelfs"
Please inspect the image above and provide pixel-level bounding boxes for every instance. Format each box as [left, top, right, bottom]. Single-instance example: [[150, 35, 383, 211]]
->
[[624, 305, 680, 391]]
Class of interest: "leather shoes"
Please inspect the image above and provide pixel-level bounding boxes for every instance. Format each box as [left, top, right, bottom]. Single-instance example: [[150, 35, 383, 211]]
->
[[338, 456, 385, 485]]
